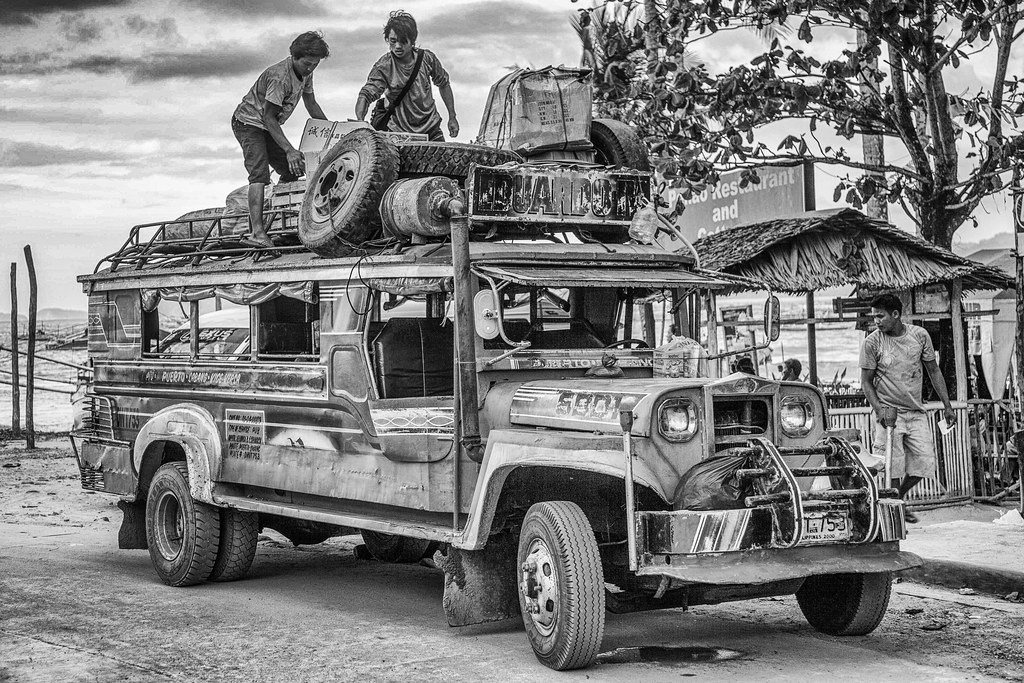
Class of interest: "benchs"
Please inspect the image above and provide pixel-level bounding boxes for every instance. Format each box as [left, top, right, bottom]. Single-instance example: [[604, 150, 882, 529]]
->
[[372, 317, 535, 399]]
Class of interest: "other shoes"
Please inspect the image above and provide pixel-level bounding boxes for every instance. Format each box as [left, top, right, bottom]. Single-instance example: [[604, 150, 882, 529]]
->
[[906, 510, 917, 523], [370, 99, 392, 131]]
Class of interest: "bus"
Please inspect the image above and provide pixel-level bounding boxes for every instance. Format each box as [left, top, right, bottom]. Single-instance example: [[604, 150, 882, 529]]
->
[[69, 162, 923, 671]]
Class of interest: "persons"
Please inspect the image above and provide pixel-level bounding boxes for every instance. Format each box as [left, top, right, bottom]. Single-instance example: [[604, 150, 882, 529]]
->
[[346, 9, 461, 146], [784, 357, 803, 383], [230, 29, 333, 250], [857, 293, 957, 524], [739, 358, 756, 373]]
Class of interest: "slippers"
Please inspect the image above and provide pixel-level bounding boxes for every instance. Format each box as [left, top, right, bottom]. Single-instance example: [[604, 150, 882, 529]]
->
[[239, 236, 280, 257]]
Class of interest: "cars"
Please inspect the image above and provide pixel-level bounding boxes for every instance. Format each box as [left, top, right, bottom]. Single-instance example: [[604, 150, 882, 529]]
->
[[151, 307, 250, 362]]
